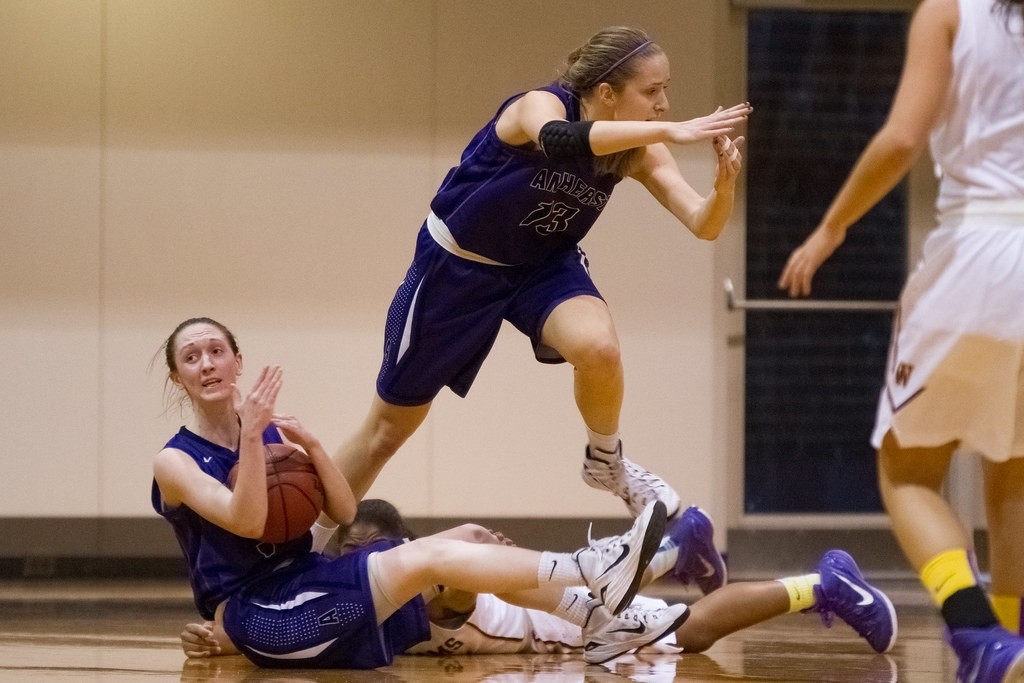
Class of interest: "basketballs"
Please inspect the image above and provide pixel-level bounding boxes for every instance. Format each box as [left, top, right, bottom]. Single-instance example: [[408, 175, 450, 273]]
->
[[221, 441, 327, 544]]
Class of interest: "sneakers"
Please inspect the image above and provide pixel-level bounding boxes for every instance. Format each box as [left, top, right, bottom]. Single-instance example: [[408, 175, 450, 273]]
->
[[852, 654, 898, 683], [582, 592, 690, 665], [943, 626, 1024, 683], [670, 505, 727, 597], [572, 499, 668, 616], [583, 664, 644, 683], [582, 439, 681, 522], [800, 550, 898, 654]]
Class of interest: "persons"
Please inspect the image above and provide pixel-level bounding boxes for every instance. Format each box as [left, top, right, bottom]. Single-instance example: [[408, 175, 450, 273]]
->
[[779, 0, 1024, 683], [151, 317, 899, 671], [310, 26, 754, 551]]
